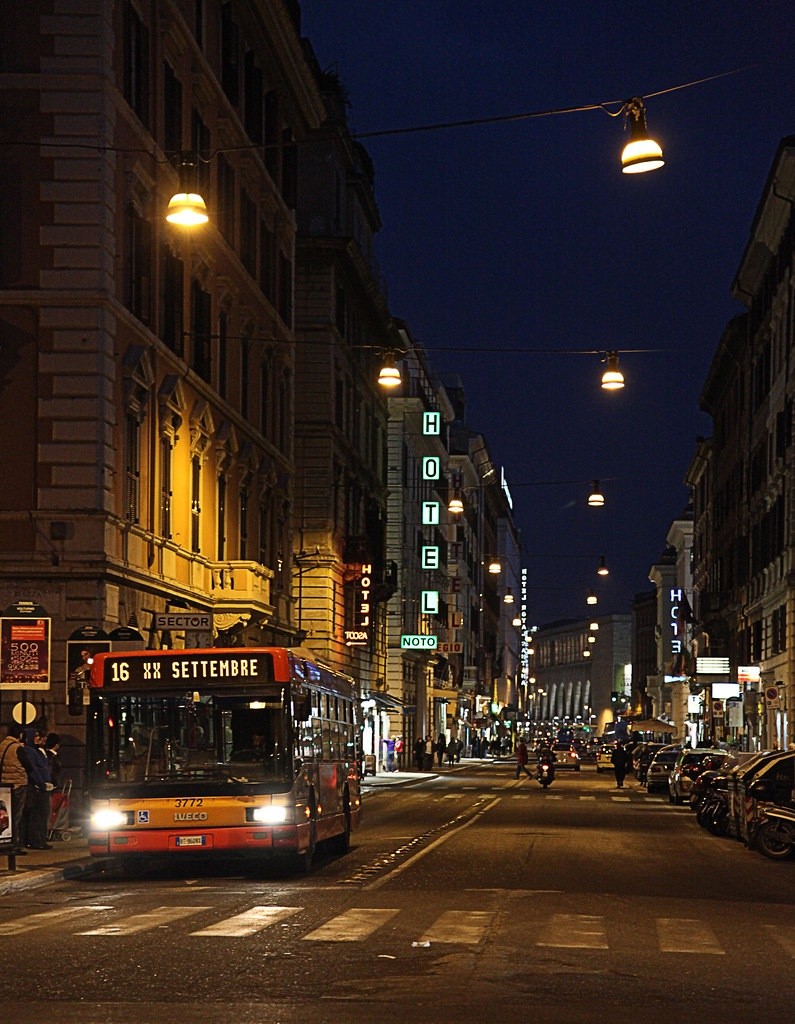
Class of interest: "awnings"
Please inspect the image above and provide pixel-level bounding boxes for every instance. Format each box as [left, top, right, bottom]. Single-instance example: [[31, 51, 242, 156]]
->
[[372, 692, 409, 708]]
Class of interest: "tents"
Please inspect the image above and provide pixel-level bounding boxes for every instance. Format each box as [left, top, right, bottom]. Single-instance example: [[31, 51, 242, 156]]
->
[[631, 717, 677, 743]]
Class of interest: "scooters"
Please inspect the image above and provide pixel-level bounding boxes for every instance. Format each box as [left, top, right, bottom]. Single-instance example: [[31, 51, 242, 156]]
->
[[748, 778, 795, 861], [680, 756, 728, 837], [536, 753, 558, 788]]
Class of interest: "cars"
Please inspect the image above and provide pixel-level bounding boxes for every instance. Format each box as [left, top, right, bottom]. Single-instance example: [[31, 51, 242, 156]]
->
[[623, 741, 681, 781], [526, 738, 552, 761], [549, 744, 581, 771], [664, 748, 727, 805], [646, 750, 681, 793], [594, 744, 624, 773], [586, 737, 602, 756]]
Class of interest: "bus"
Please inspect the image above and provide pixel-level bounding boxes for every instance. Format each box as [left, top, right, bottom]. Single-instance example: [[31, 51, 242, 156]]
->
[[66, 647, 374, 880]]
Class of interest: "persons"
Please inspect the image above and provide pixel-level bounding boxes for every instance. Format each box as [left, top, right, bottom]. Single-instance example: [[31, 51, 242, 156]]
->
[[436, 736, 456, 767], [455, 739, 464, 763], [490, 737, 501, 757], [0, 724, 62, 855], [234, 729, 273, 762], [75, 646, 97, 680], [684, 736, 729, 754], [471, 734, 489, 758], [381, 735, 404, 772], [413, 736, 425, 771], [537, 745, 558, 779], [611, 743, 628, 788], [512, 737, 533, 780], [637, 744, 653, 787], [502, 736, 507, 756], [787, 743, 795, 750], [424, 735, 436, 771]]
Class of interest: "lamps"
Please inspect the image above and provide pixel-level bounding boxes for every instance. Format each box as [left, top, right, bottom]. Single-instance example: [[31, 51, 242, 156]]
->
[[502, 587, 515, 604], [618, 96, 665, 175], [583, 647, 590, 658], [587, 632, 596, 644], [165, 150, 210, 227], [601, 353, 626, 389], [375, 346, 404, 385], [512, 612, 521, 626], [488, 556, 502, 573], [587, 589, 597, 605], [590, 615, 600, 630], [526, 632, 547, 697], [598, 557, 610, 575], [448, 488, 463, 513], [586, 479, 605, 506]]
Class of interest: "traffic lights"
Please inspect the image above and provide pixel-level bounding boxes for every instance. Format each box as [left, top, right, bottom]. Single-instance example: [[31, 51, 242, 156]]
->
[[611, 692, 617, 702]]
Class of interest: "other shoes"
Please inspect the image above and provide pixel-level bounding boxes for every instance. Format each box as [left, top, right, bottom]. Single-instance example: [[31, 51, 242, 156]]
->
[[29, 842, 53, 850], [13, 848, 28, 856], [530, 775, 534, 781], [513, 776, 519, 779]]
